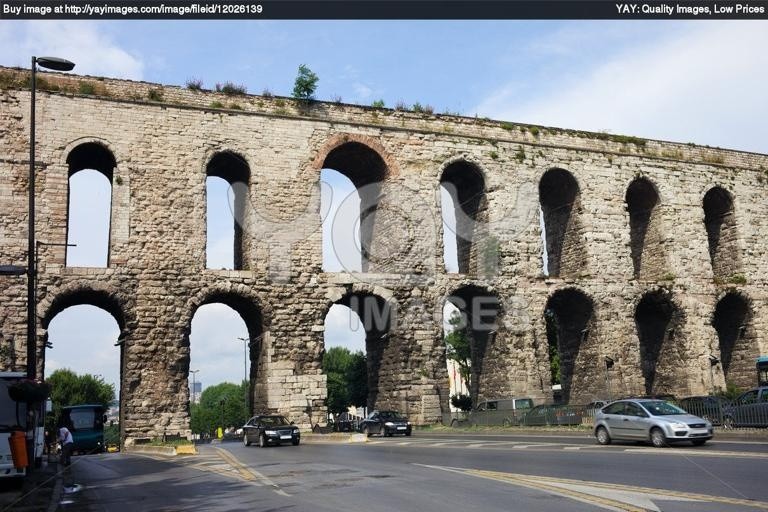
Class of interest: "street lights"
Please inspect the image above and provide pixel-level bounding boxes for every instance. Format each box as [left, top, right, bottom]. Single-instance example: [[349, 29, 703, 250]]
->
[[189, 369, 199, 404], [239, 338, 252, 421], [26, 54, 75, 496]]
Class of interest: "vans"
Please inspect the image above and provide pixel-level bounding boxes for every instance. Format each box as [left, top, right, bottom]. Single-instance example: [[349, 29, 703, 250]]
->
[[468, 399, 534, 427], [682, 388, 767, 429]]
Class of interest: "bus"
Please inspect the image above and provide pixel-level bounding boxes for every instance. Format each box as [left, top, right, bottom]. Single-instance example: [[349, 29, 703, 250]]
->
[[1, 371, 51, 480], [755, 356, 768, 386], [59, 404, 107, 456]]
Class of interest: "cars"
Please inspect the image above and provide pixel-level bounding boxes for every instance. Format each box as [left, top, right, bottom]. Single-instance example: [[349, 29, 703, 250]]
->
[[520, 402, 582, 425], [242, 415, 301, 447], [329, 408, 412, 437], [584, 399, 713, 447]]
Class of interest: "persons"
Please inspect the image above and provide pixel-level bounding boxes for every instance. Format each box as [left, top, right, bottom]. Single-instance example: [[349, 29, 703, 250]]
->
[[57, 423, 74, 467]]
